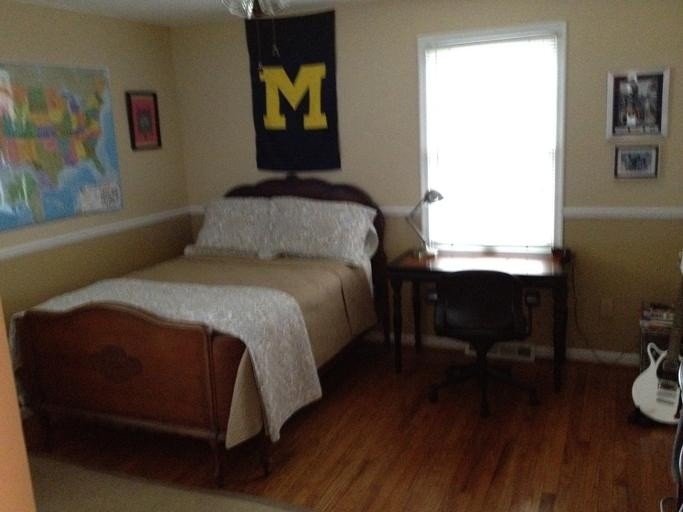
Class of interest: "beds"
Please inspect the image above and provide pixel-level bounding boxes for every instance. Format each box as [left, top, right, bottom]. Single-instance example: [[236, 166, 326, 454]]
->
[[13, 174, 384, 492]]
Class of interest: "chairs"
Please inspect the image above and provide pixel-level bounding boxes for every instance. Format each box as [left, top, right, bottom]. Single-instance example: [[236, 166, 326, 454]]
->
[[432, 270, 533, 419]]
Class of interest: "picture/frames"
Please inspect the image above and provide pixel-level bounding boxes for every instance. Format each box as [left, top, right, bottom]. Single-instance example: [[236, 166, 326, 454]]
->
[[614, 145, 660, 177], [125, 91, 163, 149], [605, 69, 671, 141]]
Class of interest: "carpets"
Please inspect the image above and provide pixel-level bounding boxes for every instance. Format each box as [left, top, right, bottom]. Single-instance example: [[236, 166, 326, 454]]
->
[[28, 457, 299, 512]]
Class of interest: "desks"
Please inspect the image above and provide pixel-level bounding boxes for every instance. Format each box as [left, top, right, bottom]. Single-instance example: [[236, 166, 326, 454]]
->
[[384, 249, 567, 395], [639, 307, 683, 371]]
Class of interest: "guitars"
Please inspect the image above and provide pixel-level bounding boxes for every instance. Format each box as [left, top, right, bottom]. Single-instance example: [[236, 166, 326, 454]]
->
[[631, 250, 683, 424]]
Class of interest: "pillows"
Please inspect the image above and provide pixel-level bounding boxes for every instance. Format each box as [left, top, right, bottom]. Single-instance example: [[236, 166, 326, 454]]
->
[[258, 196, 377, 268], [195, 196, 269, 252]]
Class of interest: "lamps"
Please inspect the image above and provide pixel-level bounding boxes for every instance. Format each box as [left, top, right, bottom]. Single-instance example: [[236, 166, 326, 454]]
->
[[405, 190, 443, 261], [221, 0, 289, 21]]
